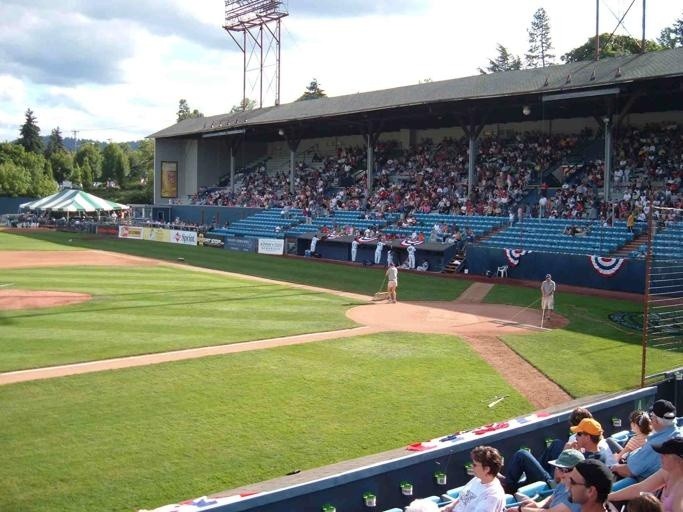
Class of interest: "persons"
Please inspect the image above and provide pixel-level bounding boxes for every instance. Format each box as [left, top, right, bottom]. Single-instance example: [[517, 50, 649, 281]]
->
[[351, 237, 361, 263], [635, 246, 653, 259], [188, 121, 682, 246], [374, 239, 429, 271], [404, 399, 682, 511], [17, 210, 188, 231], [385, 262, 398, 303], [541, 274, 555, 320], [310, 234, 321, 256]]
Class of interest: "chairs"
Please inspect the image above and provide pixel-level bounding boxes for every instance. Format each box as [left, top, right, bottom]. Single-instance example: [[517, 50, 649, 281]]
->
[[199, 127, 682, 262], [497, 265, 508, 278], [384, 414, 683, 512]]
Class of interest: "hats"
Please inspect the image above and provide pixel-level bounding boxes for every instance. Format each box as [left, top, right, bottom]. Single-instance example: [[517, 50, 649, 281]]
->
[[547, 449, 584, 469], [651, 436, 683, 455], [648, 400, 676, 419], [577, 460, 612, 492], [570, 419, 603, 436]]
